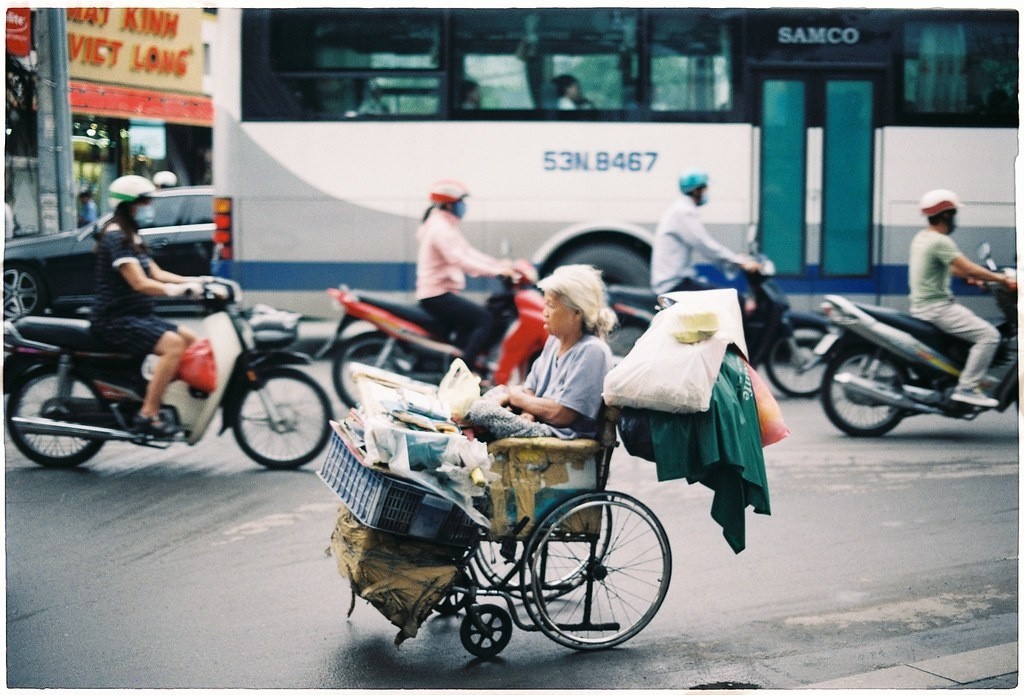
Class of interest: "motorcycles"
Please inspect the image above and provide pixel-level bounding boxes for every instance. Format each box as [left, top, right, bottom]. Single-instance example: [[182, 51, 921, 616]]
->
[[814, 242, 1020, 438], [312, 237, 552, 411], [607, 222, 834, 398], [4, 272, 336, 469]]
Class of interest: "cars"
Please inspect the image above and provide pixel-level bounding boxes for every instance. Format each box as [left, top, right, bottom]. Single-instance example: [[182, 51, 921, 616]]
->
[[4, 186, 217, 321]]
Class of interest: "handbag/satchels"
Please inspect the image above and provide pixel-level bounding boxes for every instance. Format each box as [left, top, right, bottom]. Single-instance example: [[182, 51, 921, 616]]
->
[[174, 340, 218, 394], [739, 357, 791, 446], [602, 300, 727, 412], [616, 406, 654, 462]]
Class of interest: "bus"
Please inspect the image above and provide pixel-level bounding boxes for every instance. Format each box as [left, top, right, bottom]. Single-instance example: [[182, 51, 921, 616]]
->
[[208, 8, 1020, 331]]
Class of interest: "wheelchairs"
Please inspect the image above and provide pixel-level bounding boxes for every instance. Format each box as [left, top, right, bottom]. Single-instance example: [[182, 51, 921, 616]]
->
[[430, 401, 675, 659]]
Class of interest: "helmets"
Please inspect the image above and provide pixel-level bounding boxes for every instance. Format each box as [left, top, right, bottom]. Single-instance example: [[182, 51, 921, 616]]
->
[[107, 175, 155, 215], [679, 171, 707, 194], [428, 180, 468, 206], [153, 171, 178, 186], [919, 188, 965, 216]]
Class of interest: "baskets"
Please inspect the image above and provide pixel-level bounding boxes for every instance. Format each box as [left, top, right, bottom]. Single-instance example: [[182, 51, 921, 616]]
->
[[316, 432, 488, 547]]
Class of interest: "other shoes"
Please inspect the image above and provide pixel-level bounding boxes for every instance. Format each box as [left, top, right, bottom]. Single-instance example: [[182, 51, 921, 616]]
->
[[950, 387, 1000, 407]]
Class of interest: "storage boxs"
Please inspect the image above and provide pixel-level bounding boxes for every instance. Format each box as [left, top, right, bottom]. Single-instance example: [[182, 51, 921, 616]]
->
[[316, 431, 477, 547]]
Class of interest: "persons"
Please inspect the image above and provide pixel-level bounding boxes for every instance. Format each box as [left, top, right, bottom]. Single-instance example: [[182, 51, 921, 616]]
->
[[906, 189, 1018, 407], [77, 184, 97, 227], [90, 175, 213, 433], [481, 263, 620, 439], [462, 80, 480, 110], [152, 172, 177, 189], [414, 177, 523, 370], [553, 74, 580, 109], [651, 170, 766, 310]]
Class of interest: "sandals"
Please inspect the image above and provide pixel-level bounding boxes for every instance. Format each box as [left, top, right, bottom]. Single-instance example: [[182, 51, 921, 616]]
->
[[128, 414, 186, 441]]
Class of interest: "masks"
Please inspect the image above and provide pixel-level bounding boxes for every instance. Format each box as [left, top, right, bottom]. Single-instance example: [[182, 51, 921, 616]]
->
[[451, 203, 465, 218], [698, 193, 709, 206], [125, 204, 157, 228], [951, 218, 958, 232]]
